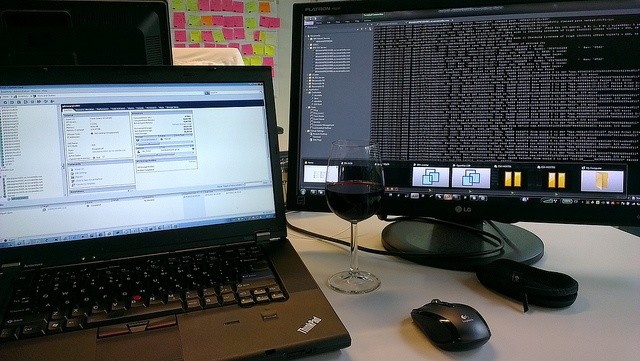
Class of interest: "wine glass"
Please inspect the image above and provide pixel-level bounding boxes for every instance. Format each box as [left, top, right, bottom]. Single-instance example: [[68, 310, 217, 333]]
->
[[326, 139, 386, 294]]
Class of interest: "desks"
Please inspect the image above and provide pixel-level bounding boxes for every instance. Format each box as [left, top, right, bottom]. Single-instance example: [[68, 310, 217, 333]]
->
[[284, 210, 640, 361]]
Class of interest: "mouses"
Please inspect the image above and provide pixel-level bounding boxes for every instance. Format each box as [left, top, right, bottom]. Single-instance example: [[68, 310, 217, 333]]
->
[[411, 299, 491, 353]]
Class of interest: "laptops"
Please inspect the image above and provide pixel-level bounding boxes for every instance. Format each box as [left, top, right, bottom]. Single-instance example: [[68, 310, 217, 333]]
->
[[0, 66, 350, 361]]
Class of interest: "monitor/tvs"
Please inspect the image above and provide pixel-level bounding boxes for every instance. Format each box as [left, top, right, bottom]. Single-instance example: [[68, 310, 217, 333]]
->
[[287, 0, 611, 271], [0, 2, 174, 65]]
